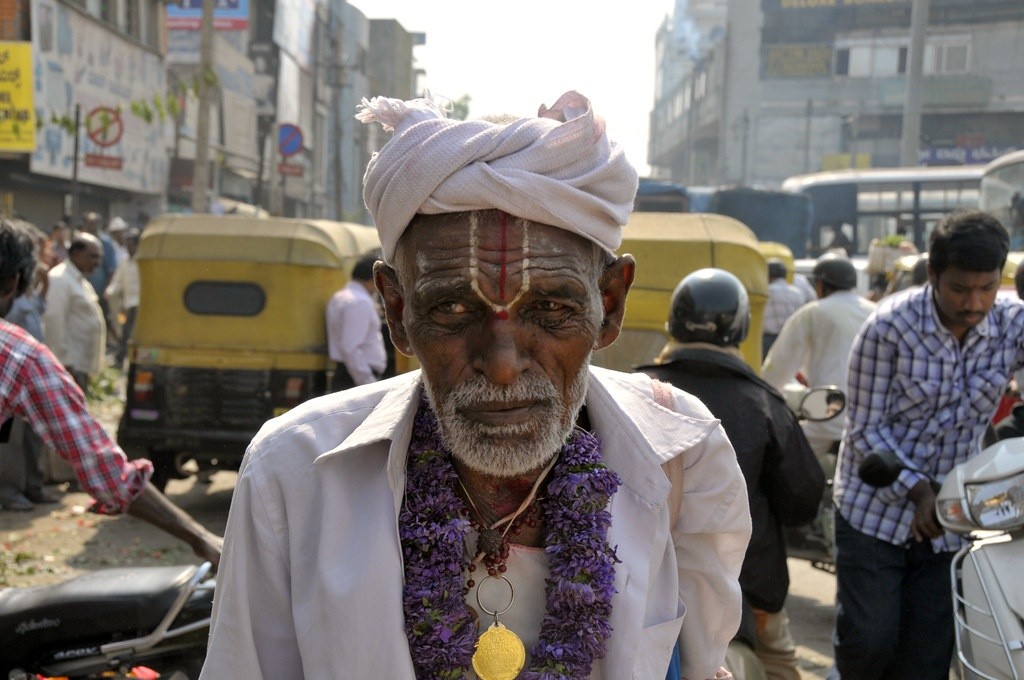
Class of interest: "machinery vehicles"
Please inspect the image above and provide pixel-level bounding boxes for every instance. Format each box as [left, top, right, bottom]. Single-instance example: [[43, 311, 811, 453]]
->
[[121, 213, 376, 487]]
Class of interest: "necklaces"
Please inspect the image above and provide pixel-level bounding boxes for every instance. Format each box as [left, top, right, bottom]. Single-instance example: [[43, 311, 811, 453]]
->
[[446, 459, 561, 678]]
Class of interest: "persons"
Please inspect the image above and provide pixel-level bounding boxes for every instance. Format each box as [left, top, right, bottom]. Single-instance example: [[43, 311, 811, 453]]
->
[[624, 264, 824, 680], [190, 87, 754, 680], [321, 254, 394, 394], [0, 202, 154, 376], [1013, 257, 1024, 302], [815, 217, 852, 252], [907, 255, 930, 289], [0, 220, 225, 572], [761, 254, 804, 368], [823, 206, 1024, 680], [753, 252, 885, 526]]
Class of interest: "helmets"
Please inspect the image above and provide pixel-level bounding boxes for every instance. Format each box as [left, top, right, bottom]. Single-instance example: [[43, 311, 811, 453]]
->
[[667, 267, 751, 347], [813, 256, 860, 288]]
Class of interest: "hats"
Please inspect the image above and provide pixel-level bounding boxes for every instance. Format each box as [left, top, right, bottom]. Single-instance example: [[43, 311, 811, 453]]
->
[[361, 87, 638, 267]]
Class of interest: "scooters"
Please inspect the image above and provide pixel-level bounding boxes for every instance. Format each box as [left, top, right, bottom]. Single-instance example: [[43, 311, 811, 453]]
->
[[864, 433, 1024, 680], [3, 558, 224, 678]]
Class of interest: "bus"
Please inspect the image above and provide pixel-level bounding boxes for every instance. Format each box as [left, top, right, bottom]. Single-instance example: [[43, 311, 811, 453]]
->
[[786, 165, 985, 255]]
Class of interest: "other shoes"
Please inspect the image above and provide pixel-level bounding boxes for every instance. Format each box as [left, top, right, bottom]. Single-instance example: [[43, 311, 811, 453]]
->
[[1, 493, 32, 509], [30, 485, 60, 503]]
